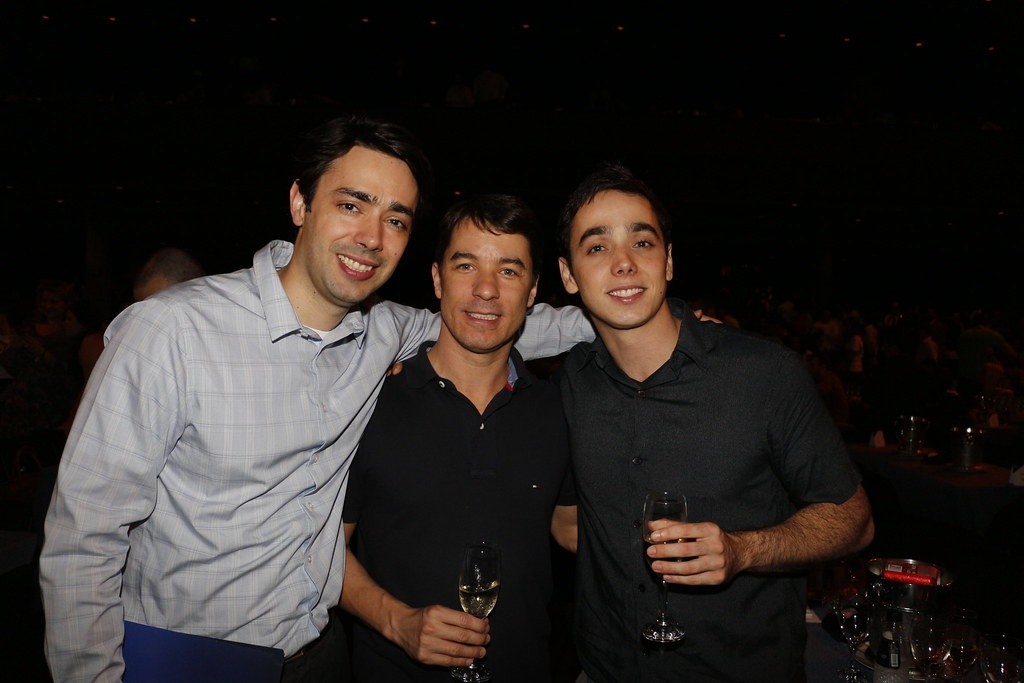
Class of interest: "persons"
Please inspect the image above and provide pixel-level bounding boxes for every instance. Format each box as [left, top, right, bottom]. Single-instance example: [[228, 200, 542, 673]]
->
[[331, 183, 579, 683], [39, 111, 728, 683], [0, 251, 203, 539], [684, 252, 1024, 429], [526, 161, 876, 683]]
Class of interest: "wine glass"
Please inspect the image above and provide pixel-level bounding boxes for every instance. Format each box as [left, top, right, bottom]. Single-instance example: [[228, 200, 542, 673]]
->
[[452, 542, 501, 683], [820, 562, 980, 683], [643, 492, 689, 643]]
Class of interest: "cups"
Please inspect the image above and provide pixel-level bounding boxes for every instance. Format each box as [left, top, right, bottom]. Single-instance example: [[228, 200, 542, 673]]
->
[[972, 393, 995, 428], [981, 633, 1024, 683], [893, 415, 928, 455], [948, 425, 986, 473]]
